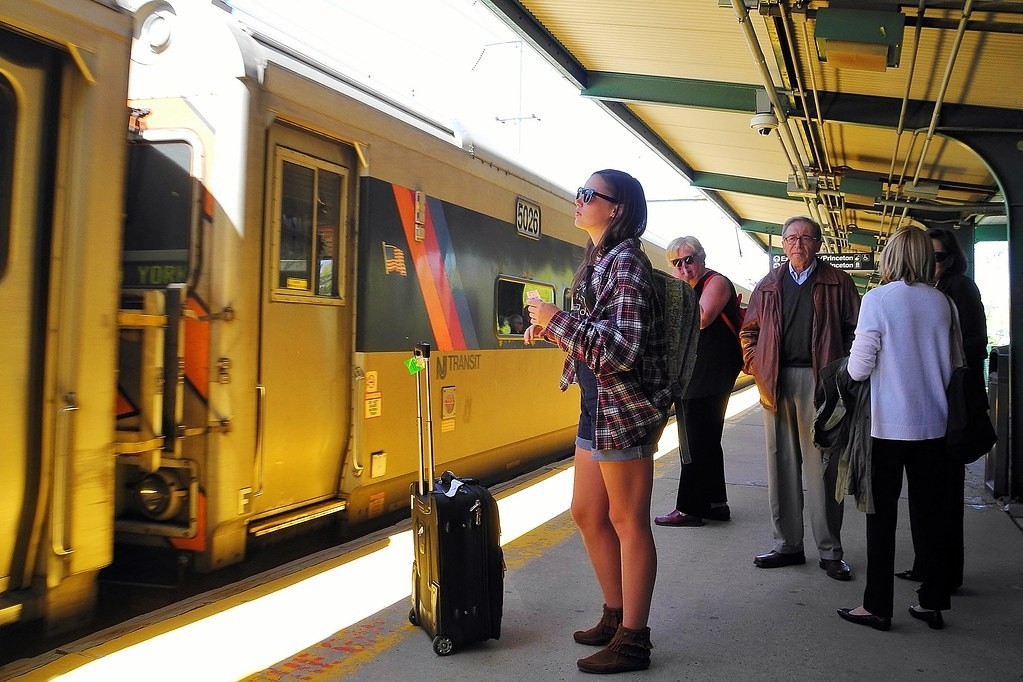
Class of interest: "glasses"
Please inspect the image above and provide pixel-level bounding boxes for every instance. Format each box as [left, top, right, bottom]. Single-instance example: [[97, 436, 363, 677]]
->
[[515, 322, 522, 325], [671, 253, 697, 267], [576, 187, 619, 204], [934, 251, 952, 262], [783, 234, 819, 245]]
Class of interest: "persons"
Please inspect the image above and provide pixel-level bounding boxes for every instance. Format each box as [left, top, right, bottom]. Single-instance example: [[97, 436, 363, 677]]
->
[[509, 315, 523, 334], [523, 168, 672, 675], [835, 225, 966, 632], [739, 217, 860, 580], [653, 236, 744, 527], [895, 228, 997, 593]]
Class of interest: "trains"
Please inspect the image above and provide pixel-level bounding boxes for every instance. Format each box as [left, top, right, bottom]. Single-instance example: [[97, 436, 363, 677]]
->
[[0, 0, 756, 644]]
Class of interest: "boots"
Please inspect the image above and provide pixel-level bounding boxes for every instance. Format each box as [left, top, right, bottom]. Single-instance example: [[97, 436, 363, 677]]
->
[[573, 602, 623, 647], [577, 624, 653, 674]]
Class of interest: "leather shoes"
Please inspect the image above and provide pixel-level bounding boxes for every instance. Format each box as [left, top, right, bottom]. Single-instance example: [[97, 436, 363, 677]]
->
[[819, 557, 852, 580], [909, 605, 944, 630], [837, 608, 892, 631], [654, 509, 703, 527], [700, 504, 732, 521], [754, 549, 806, 569]]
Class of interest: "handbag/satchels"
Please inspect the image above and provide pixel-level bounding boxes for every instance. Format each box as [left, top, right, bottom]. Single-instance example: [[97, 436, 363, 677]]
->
[[946, 294, 998, 466]]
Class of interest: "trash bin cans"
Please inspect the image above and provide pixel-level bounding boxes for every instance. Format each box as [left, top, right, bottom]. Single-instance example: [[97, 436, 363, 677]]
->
[[984, 345, 1010, 499]]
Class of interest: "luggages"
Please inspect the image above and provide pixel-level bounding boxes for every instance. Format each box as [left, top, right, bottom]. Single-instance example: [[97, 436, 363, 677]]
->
[[408, 342, 506, 656]]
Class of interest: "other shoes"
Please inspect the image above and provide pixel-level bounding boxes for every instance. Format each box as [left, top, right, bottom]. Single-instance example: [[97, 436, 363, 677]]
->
[[895, 569, 915, 580]]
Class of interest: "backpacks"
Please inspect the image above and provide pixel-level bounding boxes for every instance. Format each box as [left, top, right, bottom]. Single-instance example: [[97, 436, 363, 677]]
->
[[638, 268, 700, 402], [704, 273, 754, 377]]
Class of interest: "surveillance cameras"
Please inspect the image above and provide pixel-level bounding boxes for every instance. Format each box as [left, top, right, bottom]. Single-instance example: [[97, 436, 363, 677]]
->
[[750, 113, 779, 136]]
